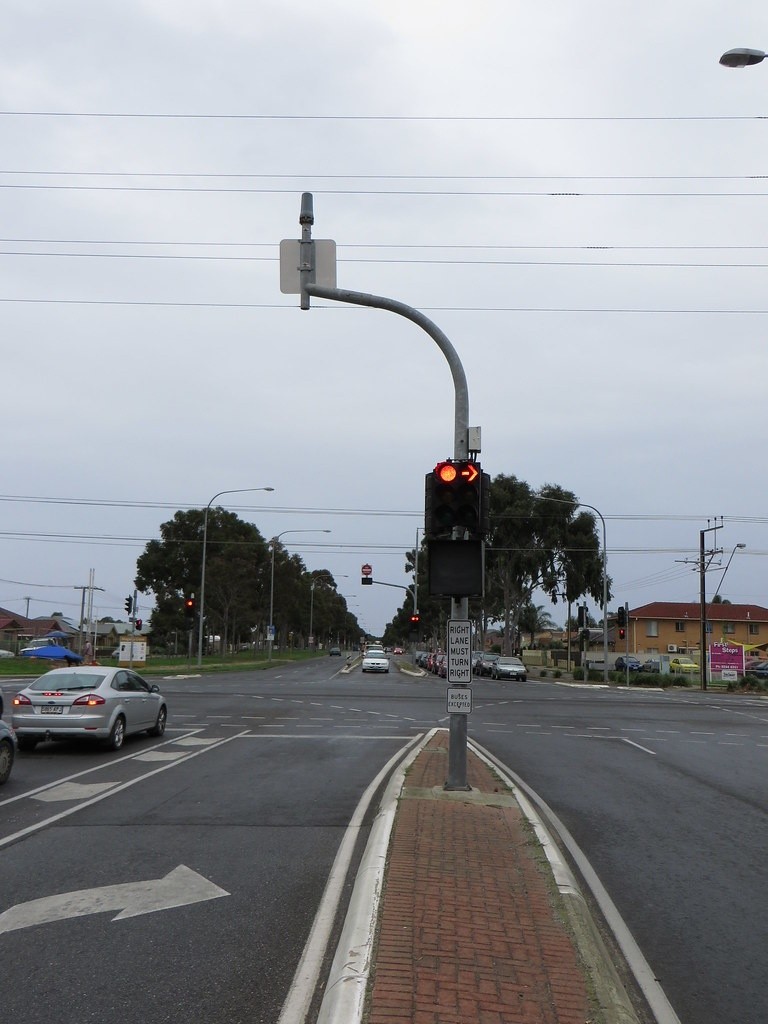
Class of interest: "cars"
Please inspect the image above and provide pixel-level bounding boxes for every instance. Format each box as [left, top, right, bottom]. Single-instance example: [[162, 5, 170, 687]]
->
[[415, 651, 528, 682], [0, 649, 14, 657], [0, 719, 18, 785], [615, 656, 642, 671], [361, 641, 403, 673], [643, 657, 659, 673], [12, 666, 168, 751], [745, 661, 768, 670], [29, 639, 56, 648], [669, 658, 701, 674], [330, 647, 341, 656]]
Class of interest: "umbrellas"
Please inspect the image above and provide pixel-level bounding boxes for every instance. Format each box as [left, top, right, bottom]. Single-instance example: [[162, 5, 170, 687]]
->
[[45, 630, 70, 645]]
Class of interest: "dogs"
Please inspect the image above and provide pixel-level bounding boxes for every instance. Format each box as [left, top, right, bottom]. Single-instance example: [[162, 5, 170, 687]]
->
[[64, 655, 83, 667]]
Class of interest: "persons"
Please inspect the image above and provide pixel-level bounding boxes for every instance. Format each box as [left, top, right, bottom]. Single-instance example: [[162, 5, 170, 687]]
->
[[84, 641, 91, 656]]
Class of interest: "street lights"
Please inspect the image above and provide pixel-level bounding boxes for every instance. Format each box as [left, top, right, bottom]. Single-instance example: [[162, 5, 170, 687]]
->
[[197, 487, 275, 667], [674, 516, 746, 690], [329, 595, 356, 650], [309, 575, 349, 653], [533, 495, 608, 683], [345, 604, 359, 650], [268, 530, 331, 660]]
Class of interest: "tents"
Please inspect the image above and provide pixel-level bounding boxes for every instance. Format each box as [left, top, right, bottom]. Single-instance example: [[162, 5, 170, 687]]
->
[[23, 645, 84, 661]]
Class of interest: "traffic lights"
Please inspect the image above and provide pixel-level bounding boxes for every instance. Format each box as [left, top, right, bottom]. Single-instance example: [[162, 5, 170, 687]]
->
[[434, 462, 481, 533], [125, 594, 132, 615], [411, 616, 419, 632], [426, 538, 486, 599], [135, 619, 142, 631], [184, 598, 195, 617], [619, 629, 625, 640], [617, 606, 626, 627]]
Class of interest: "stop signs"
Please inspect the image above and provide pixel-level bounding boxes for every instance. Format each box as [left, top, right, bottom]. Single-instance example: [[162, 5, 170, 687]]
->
[[362, 565, 372, 575]]
